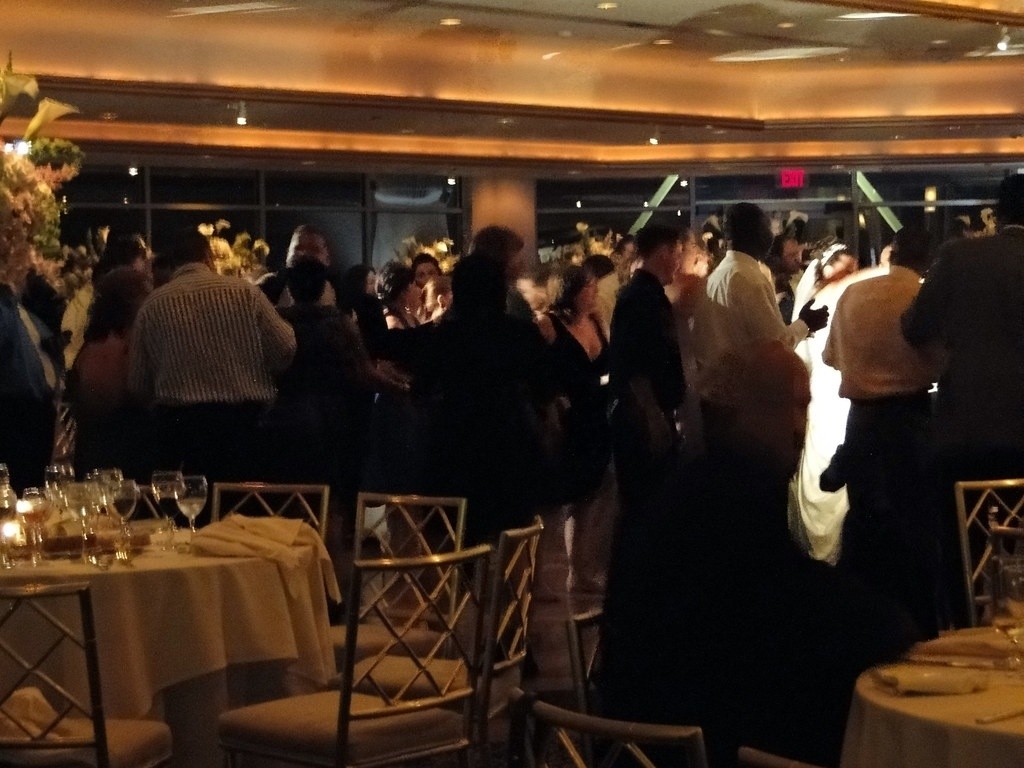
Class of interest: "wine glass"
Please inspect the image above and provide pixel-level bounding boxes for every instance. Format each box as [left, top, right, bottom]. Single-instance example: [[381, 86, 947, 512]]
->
[[0, 464, 138, 570], [988, 560, 1024, 679], [147, 471, 185, 551], [176, 477, 208, 552]]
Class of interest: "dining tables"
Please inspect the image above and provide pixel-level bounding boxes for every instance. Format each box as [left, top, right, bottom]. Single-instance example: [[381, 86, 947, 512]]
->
[[0, 527, 337, 768], [837, 626, 1024, 768]]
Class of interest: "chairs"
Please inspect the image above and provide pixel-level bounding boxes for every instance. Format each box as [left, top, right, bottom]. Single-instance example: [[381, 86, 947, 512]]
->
[[566, 607, 607, 768], [0, 580, 173, 768], [217, 543, 495, 768], [737, 746, 824, 768], [351, 514, 544, 768], [212, 481, 331, 546], [508, 685, 709, 768], [955, 478, 1024, 628], [331, 492, 468, 669]]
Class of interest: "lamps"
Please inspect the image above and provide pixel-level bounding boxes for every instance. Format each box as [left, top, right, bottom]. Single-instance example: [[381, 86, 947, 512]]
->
[[997, 24, 1011, 51]]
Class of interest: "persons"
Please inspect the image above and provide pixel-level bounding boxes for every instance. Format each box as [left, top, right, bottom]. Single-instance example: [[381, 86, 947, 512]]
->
[[0, 176, 1024, 692]]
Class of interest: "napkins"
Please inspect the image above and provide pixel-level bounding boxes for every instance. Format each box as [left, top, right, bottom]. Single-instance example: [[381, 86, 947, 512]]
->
[[913, 627, 1016, 659], [221, 511, 343, 604], [191, 520, 302, 569], [868, 665, 989, 696]]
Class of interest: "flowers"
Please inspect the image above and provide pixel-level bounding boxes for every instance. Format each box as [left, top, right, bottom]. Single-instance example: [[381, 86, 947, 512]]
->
[[198, 220, 271, 279], [0, 147, 64, 288], [571, 221, 625, 267], [392, 235, 460, 274], [23, 136, 85, 190]]
